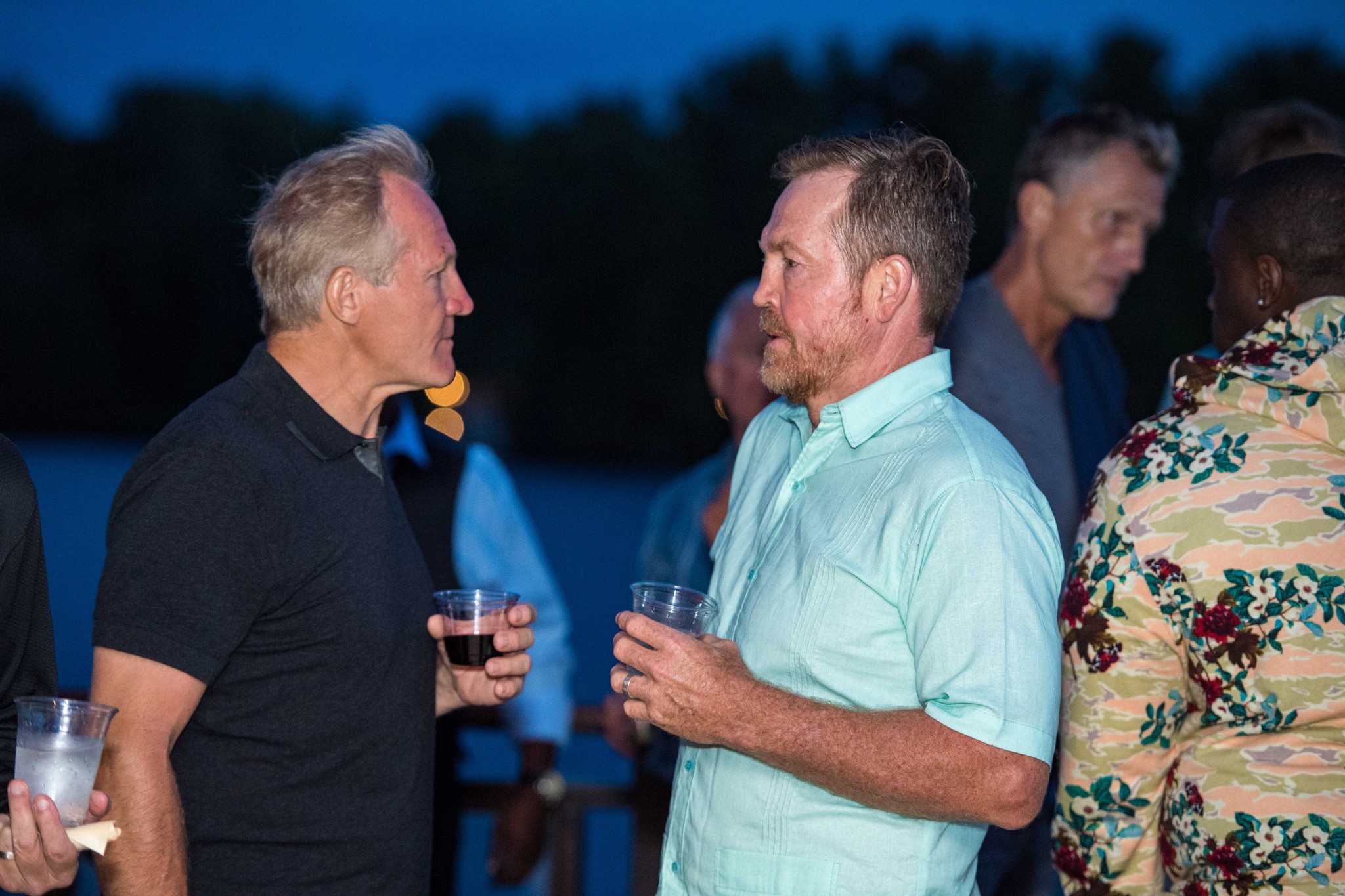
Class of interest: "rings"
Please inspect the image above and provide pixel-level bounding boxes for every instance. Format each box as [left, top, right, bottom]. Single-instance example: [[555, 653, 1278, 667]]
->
[[0, 851, 15, 859]]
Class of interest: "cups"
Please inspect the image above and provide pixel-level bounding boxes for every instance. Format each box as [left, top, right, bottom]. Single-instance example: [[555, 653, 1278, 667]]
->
[[623, 582, 720, 679], [11, 696, 119, 829], [432, 588, 521, 666]]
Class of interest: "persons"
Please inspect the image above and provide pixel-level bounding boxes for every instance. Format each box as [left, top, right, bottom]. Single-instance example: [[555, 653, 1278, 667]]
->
[[1051, 152, 1345, 896], [0, 438, 110, 896], [935, 111, 1169, 896], [611, 136, 1063, 896], [87, 131, 537, 896], [606, 292, 769, 790], [381, 404, 575, 896], [1220, 108, 1345, 174]]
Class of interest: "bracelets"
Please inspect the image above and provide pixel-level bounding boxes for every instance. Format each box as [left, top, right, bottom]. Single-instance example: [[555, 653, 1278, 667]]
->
[[520, 771, 562, 798]]
